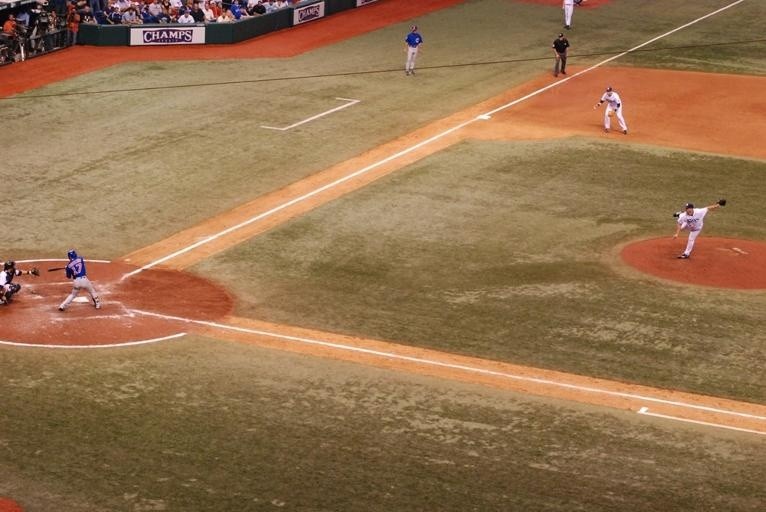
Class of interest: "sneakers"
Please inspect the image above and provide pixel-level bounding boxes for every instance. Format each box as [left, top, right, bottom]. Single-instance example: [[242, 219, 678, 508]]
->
[[95, 301, 101, 309], [677, 253, 689, 258], [406, 69, 416, 76], [605, 128, 609, 133], [564, 24, 571, 30], [58, 304, 65, 311], [673, 211, 683, 217], [624, 129, 627, 135]]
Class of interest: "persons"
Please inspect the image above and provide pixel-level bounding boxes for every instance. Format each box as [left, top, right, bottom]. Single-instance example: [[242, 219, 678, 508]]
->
[[592, 84, 628, 135], [552, 33, 570, 78], [0, 260, 40, 306], [56, 250, 101, 313], [671, 200, 724, 260], [0, 0, 95, 63], [103, 1, 288, 24], [561, 1, 577, 30], [404, 23, 423, 76]]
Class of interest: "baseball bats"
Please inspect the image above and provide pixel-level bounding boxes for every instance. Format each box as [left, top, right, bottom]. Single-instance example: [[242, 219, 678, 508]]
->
[[48, 266, 67, 272]]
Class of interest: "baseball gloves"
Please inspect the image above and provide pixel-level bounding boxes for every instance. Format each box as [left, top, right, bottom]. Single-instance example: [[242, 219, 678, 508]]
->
[[609, 112, 614, 117], [30, 268, 39, 276], [718, 199, 725, 205]]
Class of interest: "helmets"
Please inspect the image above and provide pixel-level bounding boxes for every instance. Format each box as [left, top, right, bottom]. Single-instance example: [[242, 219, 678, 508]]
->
[[4, 260, 15, 268], [67, 250, 77, 260], [410, 25, 417, 31]]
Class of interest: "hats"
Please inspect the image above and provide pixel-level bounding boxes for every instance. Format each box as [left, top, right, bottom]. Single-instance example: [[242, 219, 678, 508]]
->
[[685, 203, 694, 209], [558, 33, 564, 38], [605, 87, 612, 92]]
